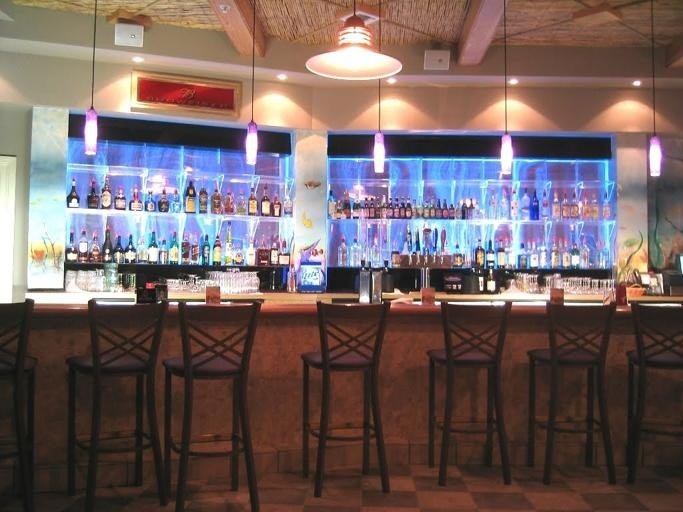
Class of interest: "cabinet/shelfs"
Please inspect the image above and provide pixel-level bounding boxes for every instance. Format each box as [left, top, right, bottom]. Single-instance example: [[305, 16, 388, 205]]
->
[[325, 217, 614, 294], [64, 206, 291, 296]]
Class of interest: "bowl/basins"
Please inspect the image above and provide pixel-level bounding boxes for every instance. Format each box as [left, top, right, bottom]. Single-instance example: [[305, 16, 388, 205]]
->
[[624, 287, 644, 298]]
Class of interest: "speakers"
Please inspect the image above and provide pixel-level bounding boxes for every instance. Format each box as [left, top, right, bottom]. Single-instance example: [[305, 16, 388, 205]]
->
[[114, 23, 144, 48], [423, 49, 450, 70]]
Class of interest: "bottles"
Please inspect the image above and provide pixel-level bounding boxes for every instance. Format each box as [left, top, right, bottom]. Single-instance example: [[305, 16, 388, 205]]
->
[[66, 220, 289, 265], [441, 273, 464, 294], [65, 177, 292, 218], [486, 268, 495, 292], [475, 270, 483, 292], [337, 236, 609, 270], [327, 189, 609, 219]]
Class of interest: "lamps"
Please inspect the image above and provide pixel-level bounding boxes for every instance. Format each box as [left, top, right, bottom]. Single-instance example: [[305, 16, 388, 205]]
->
[[370, 1, 386, 174], [80, 1, 102, 156], [646, 1, 662, 178], [243, 0, 260, 166], [302, 1, 402, 83], [497, 1, 515, 175]]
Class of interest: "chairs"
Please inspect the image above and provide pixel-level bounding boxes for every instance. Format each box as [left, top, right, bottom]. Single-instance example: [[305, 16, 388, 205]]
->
[[295, 297, 390, 497], [426, 300, 511, 489], [0, 297, 39, 511], [160, 297, 264, 512], [62, 296, 168, 510], [622, 297, 682, 484], [521, 300, 617, 487]]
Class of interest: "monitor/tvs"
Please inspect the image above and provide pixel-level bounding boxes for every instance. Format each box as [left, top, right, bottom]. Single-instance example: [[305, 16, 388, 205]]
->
[[676, 254, 683, 278]]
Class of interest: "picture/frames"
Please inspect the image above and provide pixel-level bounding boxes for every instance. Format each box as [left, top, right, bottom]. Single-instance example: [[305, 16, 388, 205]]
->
[[129, 69, 242, 121]]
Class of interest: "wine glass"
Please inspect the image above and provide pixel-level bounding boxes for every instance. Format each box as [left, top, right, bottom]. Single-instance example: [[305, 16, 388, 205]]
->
[[166, 269, 259, 293], [512, 272, 614, 295]]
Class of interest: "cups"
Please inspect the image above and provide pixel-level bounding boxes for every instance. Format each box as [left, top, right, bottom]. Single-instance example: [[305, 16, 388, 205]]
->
[[154, 284, 168, 303], [205, 286, 220, 306], [549, 287, 565, 304], [65, 266, 137, 296], [420, 287, 435, 305], [615, 286, 627, 306]]
